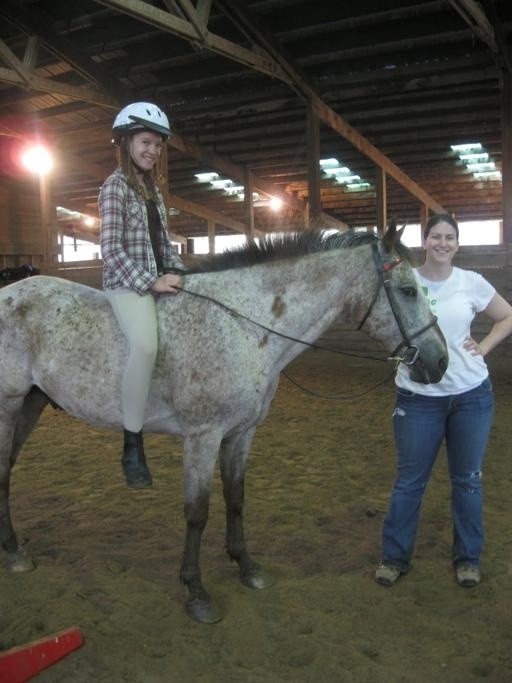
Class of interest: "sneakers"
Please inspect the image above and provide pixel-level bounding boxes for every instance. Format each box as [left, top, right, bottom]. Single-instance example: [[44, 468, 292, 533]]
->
[[375, 565, 400, 586], [456, 567, 481, 586]]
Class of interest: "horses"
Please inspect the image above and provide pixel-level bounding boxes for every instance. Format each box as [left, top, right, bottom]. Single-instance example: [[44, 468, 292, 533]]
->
[[0, 214, 449, 626]]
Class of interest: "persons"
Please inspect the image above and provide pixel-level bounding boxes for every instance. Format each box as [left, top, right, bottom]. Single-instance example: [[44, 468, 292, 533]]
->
[[374, 212, 512, 588], [97, 102, 188, 489]]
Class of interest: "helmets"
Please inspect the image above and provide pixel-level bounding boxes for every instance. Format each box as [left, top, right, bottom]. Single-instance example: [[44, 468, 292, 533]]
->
[[112, 102, 172, 136]]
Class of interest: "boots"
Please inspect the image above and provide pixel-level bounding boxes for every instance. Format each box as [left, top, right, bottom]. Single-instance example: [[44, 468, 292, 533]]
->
[[121, 429, 152, 489]]
